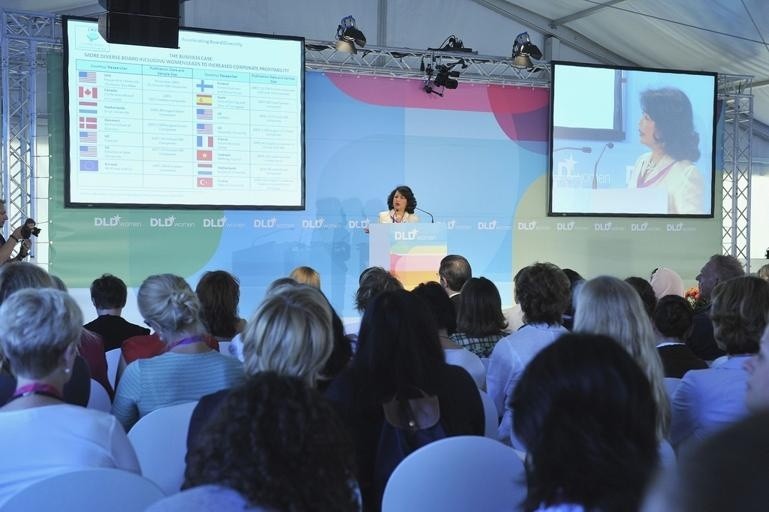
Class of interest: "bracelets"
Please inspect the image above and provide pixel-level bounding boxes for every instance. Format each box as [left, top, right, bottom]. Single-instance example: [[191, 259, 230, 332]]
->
[[19, 251, 28, 259], [9, 235, 19, 243]]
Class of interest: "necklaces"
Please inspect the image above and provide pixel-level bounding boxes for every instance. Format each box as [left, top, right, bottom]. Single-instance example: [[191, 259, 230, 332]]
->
[[646, 159, 656, 175], [438, 335, 453, 341], [395, 211, 404, 219]]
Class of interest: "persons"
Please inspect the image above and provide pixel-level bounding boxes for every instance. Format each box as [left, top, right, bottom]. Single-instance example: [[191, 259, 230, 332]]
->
[[0, 200, 37, 266], [364, 185, 421, 234], [667, 276, 768, 464], [571, 275, 670, 442], [179, 286, 376, 510], [270, 255, 504, 391], [565, 255, 746, 378], [148, 371, 362, 510], [0, 258, 250, 431], [320, 289, 487, 512], [0, 287, 143, 508], [628, 87, 705, 215], [484, 262, 571, 455], [510, 331, 665, 511], [671, 402, 768, 511]]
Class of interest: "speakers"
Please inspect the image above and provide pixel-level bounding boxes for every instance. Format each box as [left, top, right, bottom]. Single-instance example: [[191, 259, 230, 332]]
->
[[99, 12, 179, 50], [98, 0, 179, 17]]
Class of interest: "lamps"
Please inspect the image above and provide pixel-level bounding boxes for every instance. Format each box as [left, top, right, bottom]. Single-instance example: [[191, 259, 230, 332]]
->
[[332, 14, 366, 56], [510, 31, 542, 71]]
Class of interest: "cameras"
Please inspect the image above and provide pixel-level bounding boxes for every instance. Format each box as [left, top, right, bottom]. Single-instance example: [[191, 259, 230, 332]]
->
[[21, 219, 41, 241]]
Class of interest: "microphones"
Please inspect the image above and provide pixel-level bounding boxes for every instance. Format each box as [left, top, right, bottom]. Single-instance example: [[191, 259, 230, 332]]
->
[[552, 146, 592, 153], [592, 142, 614, 189], [393, 209, 398, 223], [412, 205, 434, 223]]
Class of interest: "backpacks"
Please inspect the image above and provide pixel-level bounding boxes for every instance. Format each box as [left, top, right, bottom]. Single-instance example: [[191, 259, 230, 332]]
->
[[372, 384, 452, 480]]
